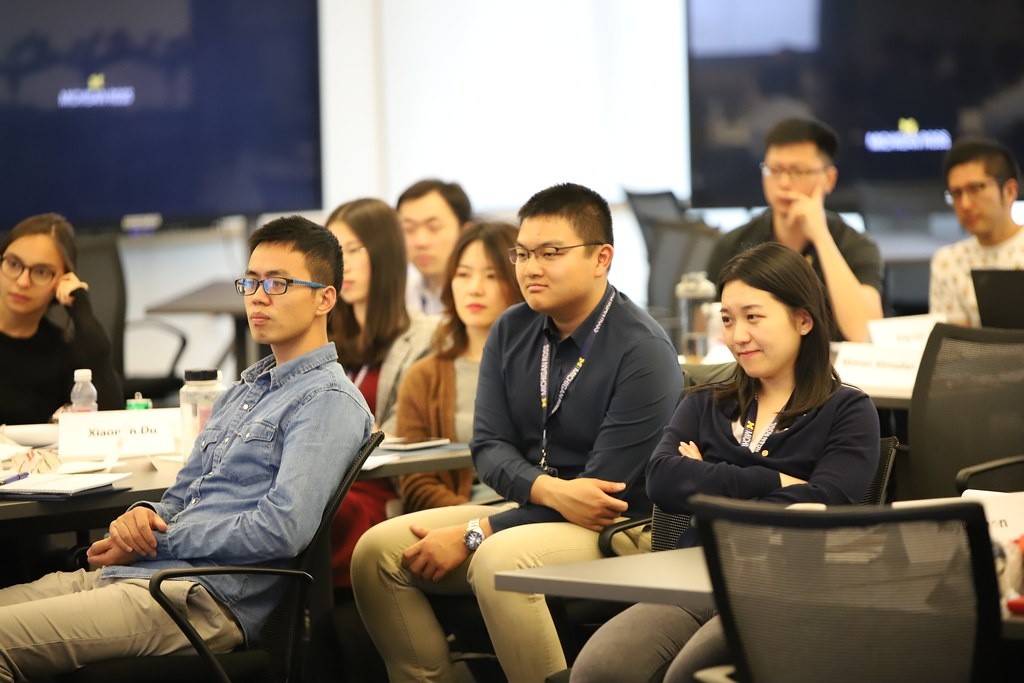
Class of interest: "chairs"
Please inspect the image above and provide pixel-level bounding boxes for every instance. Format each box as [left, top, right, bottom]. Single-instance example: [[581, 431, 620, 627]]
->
[[64, 186, 1024, 682]]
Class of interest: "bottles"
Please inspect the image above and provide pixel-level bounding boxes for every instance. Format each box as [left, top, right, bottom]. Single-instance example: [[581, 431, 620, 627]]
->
[[178, 371, 224, 447], [675, 271, 717, 360], [70, 369, 99, 412]]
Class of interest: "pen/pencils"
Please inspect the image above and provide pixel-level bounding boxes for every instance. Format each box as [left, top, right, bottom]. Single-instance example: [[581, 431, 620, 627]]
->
[[0, 472, 29, 486]]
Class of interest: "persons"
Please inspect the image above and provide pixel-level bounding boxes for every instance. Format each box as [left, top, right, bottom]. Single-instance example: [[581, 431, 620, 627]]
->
[[565, 245, 879, 683], [0, 216, 376, 683], [321, 179, 685, 683], [0, 211, 124, 426], [930, 142, 1024, 329], [706, 120, 886, 345]]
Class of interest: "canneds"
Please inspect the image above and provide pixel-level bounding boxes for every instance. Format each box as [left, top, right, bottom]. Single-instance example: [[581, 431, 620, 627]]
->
[[125, 392, 153, 410]]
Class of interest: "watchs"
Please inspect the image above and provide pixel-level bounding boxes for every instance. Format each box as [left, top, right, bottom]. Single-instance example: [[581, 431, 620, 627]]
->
[[463, 518, 485, 551]]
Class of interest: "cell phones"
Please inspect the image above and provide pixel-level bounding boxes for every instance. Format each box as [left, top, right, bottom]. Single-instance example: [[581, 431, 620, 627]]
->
[[380, 438, 450, 450]]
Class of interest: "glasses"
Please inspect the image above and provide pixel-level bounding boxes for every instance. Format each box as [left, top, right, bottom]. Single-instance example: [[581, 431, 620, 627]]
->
[[760, 161, 827, 183], [0, 256, 56, 287], [944, 180, 1000, 206], [507, 241, 605, 265], [234, 276, 326, 296]]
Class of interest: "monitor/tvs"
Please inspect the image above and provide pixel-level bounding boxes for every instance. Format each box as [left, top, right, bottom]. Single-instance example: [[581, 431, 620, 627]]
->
[[685, 0, 1024, 212], [0, 0, 326, 237]]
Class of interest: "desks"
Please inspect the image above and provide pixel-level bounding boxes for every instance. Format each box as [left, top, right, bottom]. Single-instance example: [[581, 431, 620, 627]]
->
[[1, 406, 476, 683], [492, 490, 1023, 642], [665, 325, 947, 407]]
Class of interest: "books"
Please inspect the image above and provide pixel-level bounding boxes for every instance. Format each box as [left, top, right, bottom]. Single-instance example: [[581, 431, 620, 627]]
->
[[0, 472, 134, 500]]
